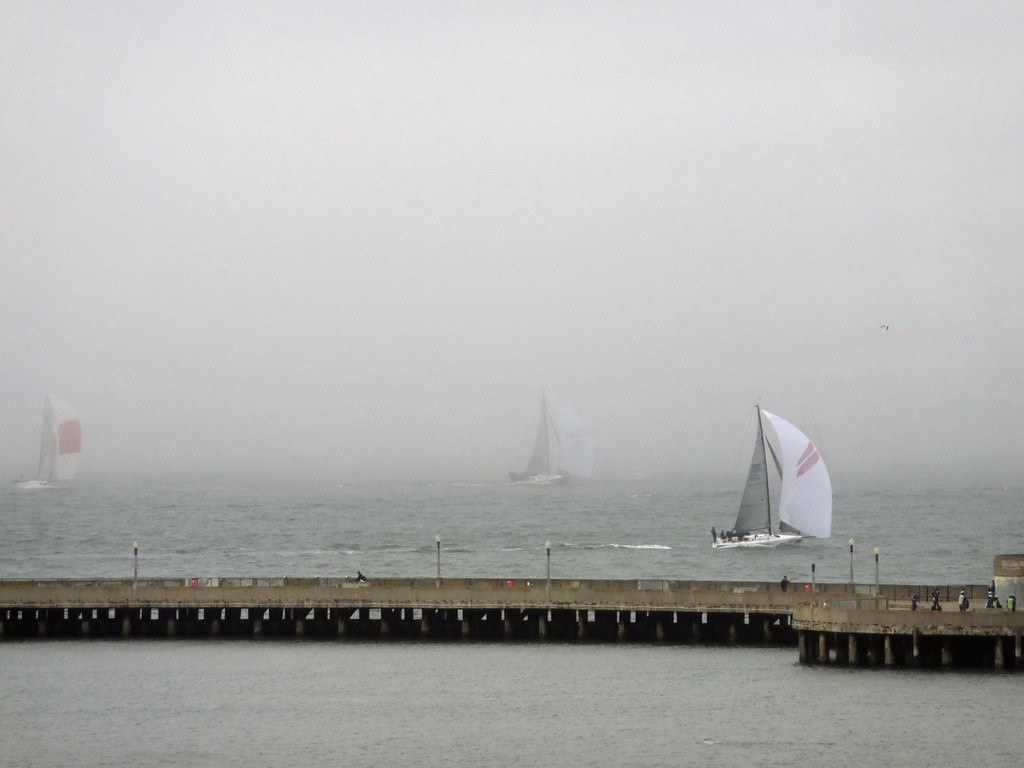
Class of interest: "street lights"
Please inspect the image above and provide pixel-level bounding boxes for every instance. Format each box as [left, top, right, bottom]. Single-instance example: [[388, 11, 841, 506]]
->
[[872, 546, 881, 598], [847, 536, 856, 584], [131, 539, 140, 586], [435, 532, 443, 586], [811, 561, 816, 593], [545, 539, 553, 587]]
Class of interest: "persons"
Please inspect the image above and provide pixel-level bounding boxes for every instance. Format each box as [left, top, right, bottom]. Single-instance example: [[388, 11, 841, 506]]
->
[[780, 576, 791, 592], [911, 592, 920, 610], [931, 588, 943, 611], [1006, 594, 1017, 612], [711, 527, 735, 545], [958, 590, 970, 612]]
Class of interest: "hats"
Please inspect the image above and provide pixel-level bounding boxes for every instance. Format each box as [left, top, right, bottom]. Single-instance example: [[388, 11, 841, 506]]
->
[[961, 591, 965, 594]]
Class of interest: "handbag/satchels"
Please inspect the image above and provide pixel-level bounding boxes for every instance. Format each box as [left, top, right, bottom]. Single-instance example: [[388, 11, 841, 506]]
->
[[963, 597, 969, 608]]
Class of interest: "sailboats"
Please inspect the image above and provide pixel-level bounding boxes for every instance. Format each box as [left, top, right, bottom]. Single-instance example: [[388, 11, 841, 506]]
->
[[521, 390, 567, 486], [712, 403, 833, 548], [9, 388, 62, 491]]
